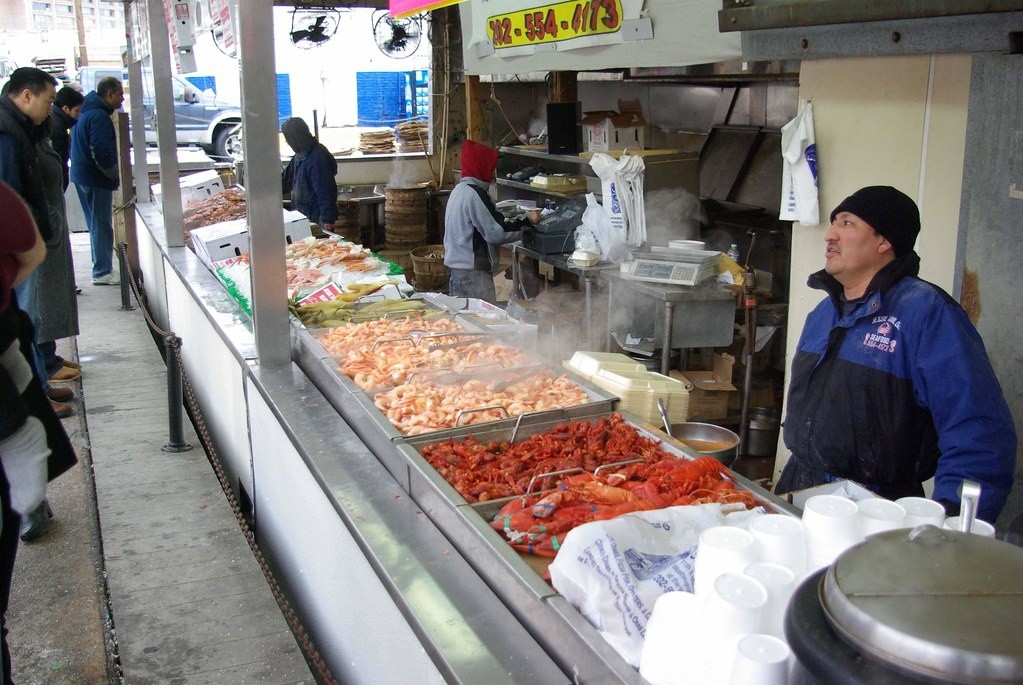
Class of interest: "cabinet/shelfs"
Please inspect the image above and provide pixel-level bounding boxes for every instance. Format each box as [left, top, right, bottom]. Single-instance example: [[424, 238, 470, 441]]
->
[[601, 270, 757, 425], [495, 147, 702, 351]]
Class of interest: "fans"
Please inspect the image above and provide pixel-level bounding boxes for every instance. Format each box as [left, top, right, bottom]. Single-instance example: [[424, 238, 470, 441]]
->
[[372, 7, 422, 59], [290, 7, 341, 50]]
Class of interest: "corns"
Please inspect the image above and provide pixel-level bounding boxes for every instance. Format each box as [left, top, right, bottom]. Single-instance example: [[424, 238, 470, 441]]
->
[[296, 298, 441, 328]]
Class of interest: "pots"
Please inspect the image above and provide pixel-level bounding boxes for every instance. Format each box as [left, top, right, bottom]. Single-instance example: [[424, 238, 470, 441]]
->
[[783, 524, 1023, 685]]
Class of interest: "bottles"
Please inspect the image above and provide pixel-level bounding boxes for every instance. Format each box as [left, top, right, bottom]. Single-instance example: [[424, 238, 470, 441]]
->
[[727, 244, 739, 265]]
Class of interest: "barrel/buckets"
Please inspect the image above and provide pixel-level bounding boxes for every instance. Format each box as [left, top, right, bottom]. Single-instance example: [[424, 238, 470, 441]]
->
[[668, 240, 705, 250], [739, 407, 782, 456], [376, 183, 451, 295]]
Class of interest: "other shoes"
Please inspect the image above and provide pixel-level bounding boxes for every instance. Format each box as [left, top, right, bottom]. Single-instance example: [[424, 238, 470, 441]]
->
[[92, 268, 122, 285], [47, 386, 74, 401], [47, 397, 73, 417], [48, 359, 82, 382]]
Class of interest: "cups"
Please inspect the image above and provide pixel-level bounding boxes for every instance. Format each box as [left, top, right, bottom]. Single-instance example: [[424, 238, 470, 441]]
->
[[639, 495, 994, 685]]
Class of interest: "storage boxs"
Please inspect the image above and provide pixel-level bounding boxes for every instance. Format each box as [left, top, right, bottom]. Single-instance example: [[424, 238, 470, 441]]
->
[[669, 333, 774, 419], [507, 292, 596, 353], [776, 480, 883, 511], [576, 98, 648, 152], [151, 169, 225, 211], [587, 176, 602, 195], [190, 207, 312, 270], [432, 297, 538, 352]]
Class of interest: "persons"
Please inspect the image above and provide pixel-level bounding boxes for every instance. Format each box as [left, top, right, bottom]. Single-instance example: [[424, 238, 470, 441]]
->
[[68, 77, 125, 286], [774, 186, 1018, 527], [0, 67, 86, 685], [443, 140, 545, 309], [280, 117, 341, 232]]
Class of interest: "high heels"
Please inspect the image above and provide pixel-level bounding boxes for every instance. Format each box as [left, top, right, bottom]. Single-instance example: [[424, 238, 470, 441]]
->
[[20, 497, 53, 541]]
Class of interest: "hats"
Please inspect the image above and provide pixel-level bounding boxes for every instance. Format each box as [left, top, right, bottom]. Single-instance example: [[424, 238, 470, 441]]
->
[[831, 185, 921, 262]]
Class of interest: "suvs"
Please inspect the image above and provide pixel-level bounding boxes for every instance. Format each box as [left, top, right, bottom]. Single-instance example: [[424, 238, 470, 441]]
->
[[67, 65, 242, 166]]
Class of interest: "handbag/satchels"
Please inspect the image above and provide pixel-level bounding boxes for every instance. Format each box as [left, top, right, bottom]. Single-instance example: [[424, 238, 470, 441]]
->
[[547, 500, 771, 672], [572, 192, 637, 265]]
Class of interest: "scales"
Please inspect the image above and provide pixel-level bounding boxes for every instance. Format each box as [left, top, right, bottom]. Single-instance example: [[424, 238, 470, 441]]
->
[[624, 249, 726, 289]]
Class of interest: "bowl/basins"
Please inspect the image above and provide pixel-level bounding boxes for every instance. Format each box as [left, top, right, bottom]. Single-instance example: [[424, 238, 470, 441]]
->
[[561, 349, 690, 429], [657, 422, 740, 467]]
